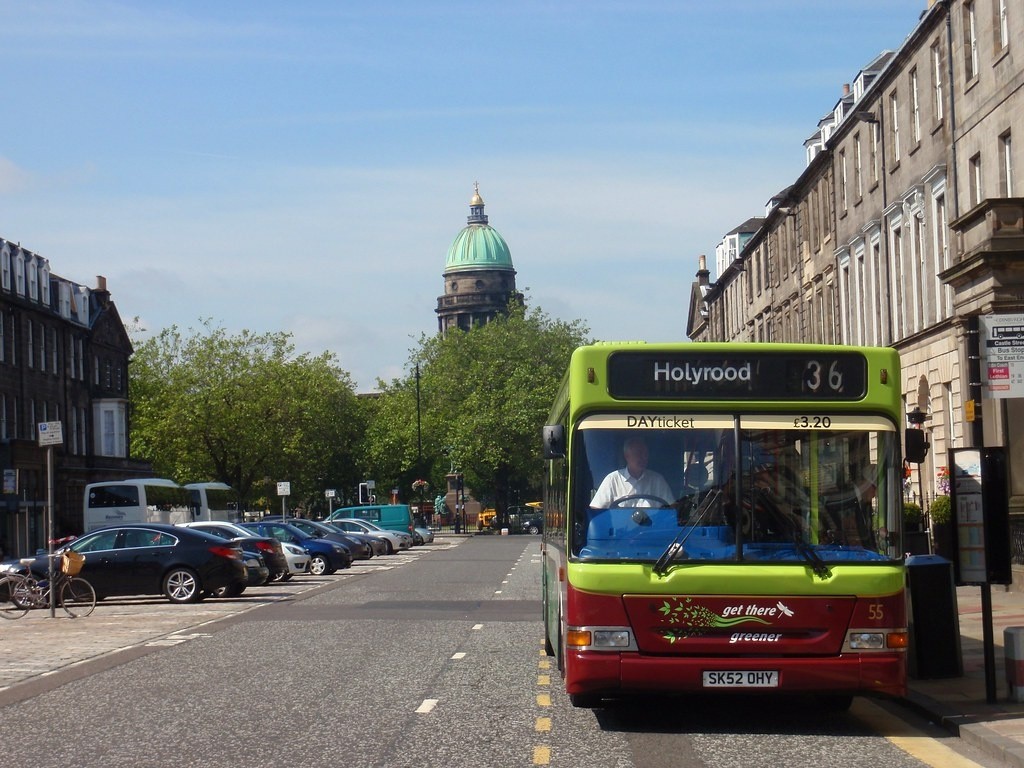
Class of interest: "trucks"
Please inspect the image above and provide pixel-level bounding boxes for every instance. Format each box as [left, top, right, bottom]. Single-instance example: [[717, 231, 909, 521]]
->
[[477, 505, 499, 530]]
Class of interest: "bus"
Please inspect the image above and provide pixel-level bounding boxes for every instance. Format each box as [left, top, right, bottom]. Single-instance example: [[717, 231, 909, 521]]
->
[[542, 341, 926, 722], [83, 478, 194, 535], [182, 482, 239, 523]]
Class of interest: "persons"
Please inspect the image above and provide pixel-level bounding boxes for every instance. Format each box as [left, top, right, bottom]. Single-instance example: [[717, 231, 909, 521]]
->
[[814, 463, 879, 546], [588, 434, 676, 510], [677, 463, 728, 528]]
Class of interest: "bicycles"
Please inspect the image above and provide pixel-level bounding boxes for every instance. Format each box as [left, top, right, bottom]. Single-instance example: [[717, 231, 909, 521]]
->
[[0, 543, 96, 620]]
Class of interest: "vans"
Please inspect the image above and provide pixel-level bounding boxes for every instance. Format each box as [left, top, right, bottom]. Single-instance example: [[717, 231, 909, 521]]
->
[[322, 505, 415, 549]]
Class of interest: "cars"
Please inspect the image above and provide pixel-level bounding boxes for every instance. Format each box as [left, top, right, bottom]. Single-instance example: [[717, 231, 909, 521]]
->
[[184, 525, 289, 587], [238, 522, 349, 575], [493, 506, 545, 527], [322, 520, 435, 556], [171, 521, 311, 583], [267, 519, 374, 569], [519, 513, 544, 535], [210, 537, 269, 597], [314, 522, 388, 561], [0, 523, 249, 610]]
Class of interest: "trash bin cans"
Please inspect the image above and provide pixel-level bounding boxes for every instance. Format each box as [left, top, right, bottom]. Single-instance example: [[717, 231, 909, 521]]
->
[[903, 554, 964, 679]]
